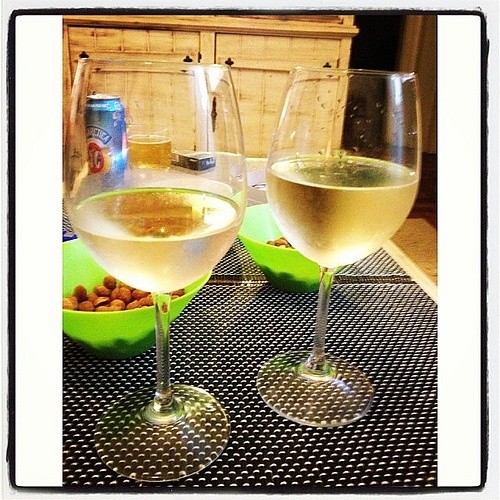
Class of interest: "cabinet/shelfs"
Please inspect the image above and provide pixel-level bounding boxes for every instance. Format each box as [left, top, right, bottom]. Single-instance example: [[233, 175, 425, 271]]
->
[[63, 15, 360, 158]]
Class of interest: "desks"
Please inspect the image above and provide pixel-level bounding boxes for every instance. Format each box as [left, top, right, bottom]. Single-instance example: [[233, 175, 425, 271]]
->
[[62, 199, 438, 487]]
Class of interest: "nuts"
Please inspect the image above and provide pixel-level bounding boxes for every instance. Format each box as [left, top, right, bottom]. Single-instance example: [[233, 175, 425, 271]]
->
[[63, 276, 184, 311], [267, 236, 291, 248]]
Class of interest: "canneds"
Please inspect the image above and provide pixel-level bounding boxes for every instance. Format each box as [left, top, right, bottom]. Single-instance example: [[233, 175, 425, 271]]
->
[[83, 94, 128, 190]]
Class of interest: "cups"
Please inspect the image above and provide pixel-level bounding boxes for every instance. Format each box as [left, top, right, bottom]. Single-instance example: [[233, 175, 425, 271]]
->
[[121, 92, 176, 180]]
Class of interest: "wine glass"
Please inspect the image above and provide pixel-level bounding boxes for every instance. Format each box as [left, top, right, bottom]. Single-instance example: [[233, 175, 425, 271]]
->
[[256, 67, 421, 426], [62, 58, 248, 481]]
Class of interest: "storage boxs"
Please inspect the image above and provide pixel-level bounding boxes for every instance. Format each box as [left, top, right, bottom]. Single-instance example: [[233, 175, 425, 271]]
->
[[172, 150, 216, 171]]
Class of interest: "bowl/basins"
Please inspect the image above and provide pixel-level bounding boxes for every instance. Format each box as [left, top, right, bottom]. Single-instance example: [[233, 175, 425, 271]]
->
[[62, 237, 213, 359], [237, 203, 346, 291]]
